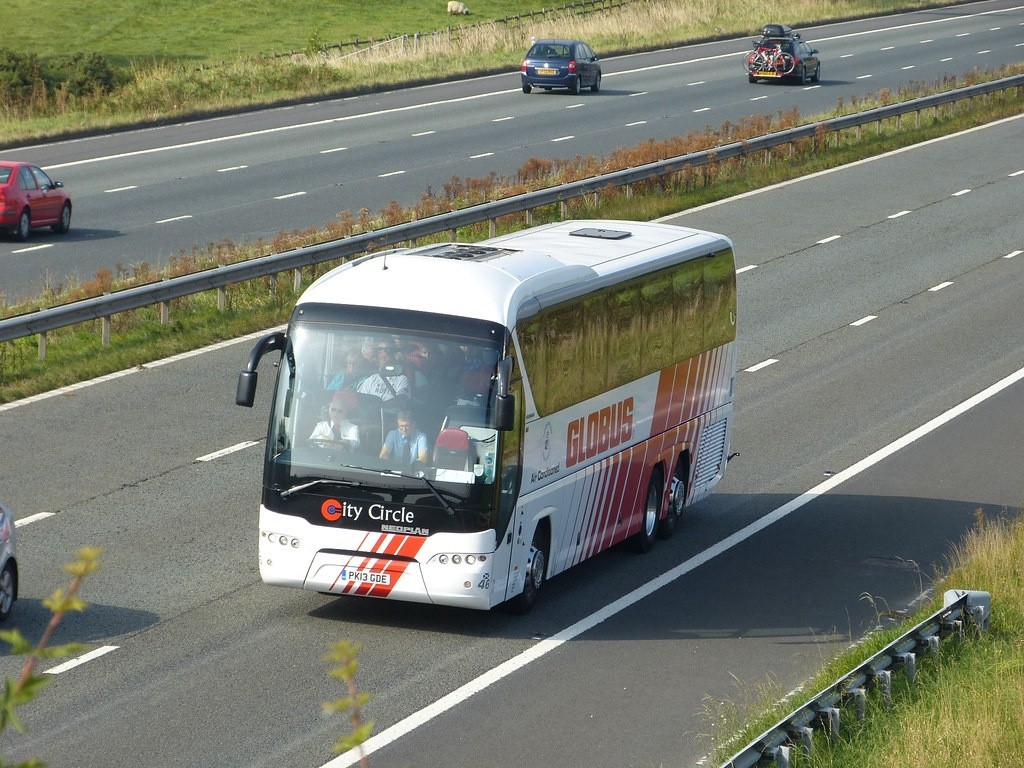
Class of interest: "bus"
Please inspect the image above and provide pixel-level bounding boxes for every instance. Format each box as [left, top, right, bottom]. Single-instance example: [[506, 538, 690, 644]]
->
[[235, 219, 738, 619]]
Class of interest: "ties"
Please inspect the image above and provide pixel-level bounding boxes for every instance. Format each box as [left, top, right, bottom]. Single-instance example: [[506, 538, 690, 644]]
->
[[332, 424, 340, 439], [402, 438, 410, 470]]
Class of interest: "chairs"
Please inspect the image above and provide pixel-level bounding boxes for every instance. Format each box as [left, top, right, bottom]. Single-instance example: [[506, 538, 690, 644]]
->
[[330, 351, 494, 472]]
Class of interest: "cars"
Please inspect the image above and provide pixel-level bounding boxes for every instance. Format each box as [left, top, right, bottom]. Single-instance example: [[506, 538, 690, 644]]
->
[[0, 160, 72, 243], [0, 505, 21, 623], [521, 40, 603, 95]]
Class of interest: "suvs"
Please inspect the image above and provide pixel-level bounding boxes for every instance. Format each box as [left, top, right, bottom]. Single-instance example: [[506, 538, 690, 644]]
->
[[749, 25, 821, 86]]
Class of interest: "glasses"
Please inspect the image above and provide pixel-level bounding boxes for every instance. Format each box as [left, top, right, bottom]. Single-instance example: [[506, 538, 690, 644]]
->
[[330, 407, 343, 414]]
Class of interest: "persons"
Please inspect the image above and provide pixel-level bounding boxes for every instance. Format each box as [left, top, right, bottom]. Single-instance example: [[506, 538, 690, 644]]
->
[[380, 410, 429, 467], [307, 398, 361, 454], [325, 334, 484, 406]]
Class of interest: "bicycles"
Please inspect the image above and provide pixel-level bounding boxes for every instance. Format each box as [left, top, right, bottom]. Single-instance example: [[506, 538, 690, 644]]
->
[[742, 40, 795, 75]]
